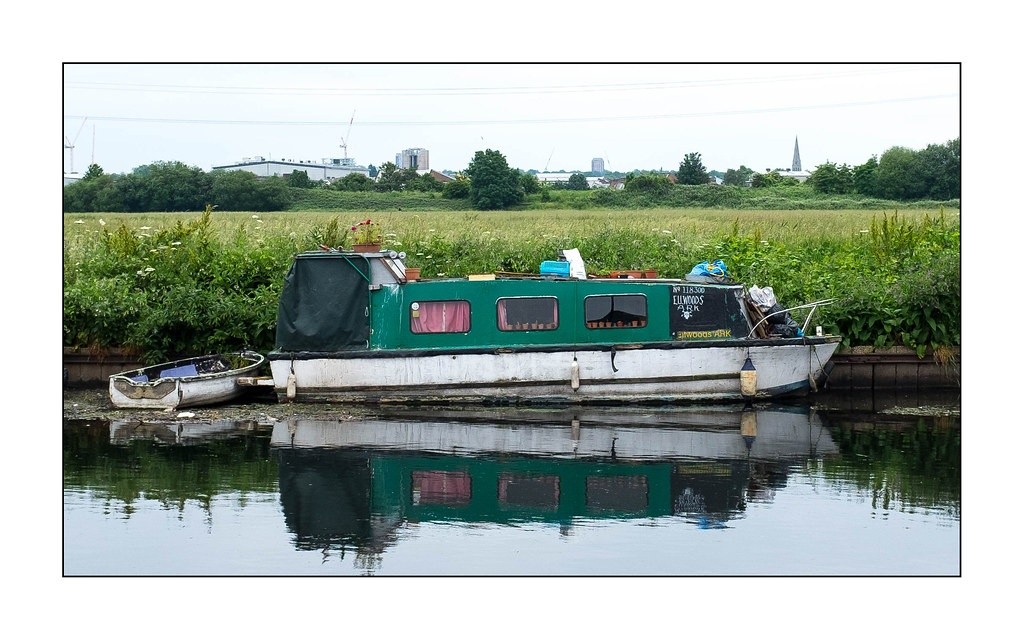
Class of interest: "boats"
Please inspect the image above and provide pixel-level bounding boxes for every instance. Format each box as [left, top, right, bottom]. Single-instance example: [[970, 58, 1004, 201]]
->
[[267, 393, 843, 557], [107, 348, 266, 411], [268, 219, 845, 404]]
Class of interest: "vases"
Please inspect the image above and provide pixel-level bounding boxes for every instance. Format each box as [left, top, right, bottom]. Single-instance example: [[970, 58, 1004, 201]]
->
[[507, 320, 557, 331], [587, 320, 645, 327]]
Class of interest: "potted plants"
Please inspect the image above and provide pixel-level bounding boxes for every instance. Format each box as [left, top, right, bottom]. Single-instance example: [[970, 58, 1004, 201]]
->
[[405, 263, 420, 279], [598, 263, 659, 278]]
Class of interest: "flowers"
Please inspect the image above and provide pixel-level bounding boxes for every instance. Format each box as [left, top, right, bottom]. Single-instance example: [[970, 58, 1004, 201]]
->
[[352, 220, 382, 244], [351, 244, 380, 253]]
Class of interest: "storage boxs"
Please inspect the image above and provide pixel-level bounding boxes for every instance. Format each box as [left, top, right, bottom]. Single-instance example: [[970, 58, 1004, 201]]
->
[[540, 260, 570, 279]]
